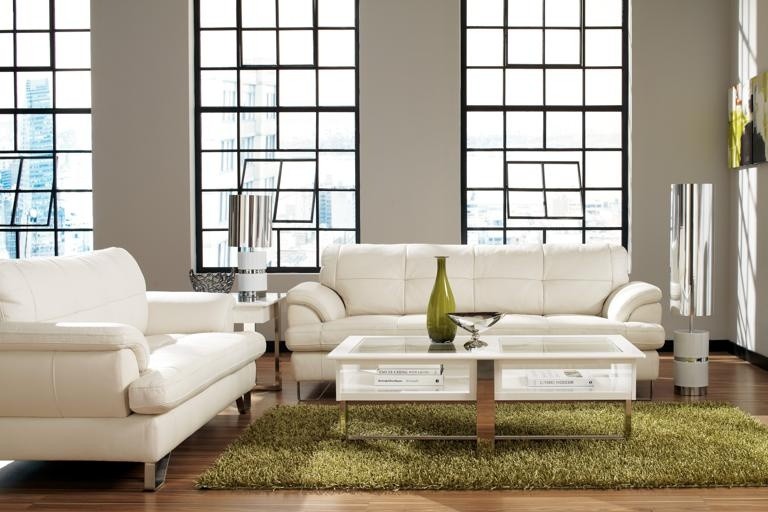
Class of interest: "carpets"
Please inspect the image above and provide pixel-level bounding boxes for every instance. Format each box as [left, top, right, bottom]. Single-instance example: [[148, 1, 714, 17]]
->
[[200, 402, 765, 491]]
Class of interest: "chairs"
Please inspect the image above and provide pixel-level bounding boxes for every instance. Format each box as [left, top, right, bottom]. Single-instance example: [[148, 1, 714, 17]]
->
[[1, 244, 265, 491]]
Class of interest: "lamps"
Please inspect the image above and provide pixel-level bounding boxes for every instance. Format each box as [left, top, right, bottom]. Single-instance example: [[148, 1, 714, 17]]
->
[[668, 182, 716, 394], [225, 195, 274, 293]]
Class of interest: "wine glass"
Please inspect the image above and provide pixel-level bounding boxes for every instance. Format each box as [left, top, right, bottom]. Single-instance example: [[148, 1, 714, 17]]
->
[[447, 312, 509, 351]]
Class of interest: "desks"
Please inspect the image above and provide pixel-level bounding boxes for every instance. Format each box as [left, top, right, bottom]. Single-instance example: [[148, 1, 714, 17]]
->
[[228, 293, 290, 391]]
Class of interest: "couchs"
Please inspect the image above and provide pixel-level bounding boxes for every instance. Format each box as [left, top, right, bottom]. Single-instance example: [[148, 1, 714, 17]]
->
[[284, 242, 663, 402]]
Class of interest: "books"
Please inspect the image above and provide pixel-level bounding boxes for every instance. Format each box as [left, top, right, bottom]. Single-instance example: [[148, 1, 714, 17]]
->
[[527, 369, 593, 386], [377, 369, 444, 375], [374, 376, 444, 386], [378, 364, 442, 369]]
